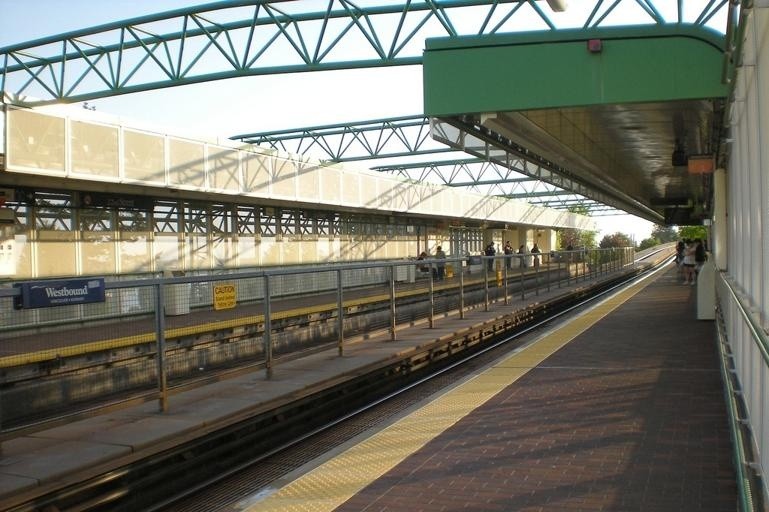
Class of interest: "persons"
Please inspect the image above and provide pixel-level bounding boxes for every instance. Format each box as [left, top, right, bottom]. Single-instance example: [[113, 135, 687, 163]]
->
[[434, 246, 446, 281], [518, 245, 525, 268], [415, 251, 437, 280], [504, 240, 513, 270], [485, 242, 498, 272], [675, 237, 708, 286], [566, 245, 573, 252], [531, 243, 540, 267]]
